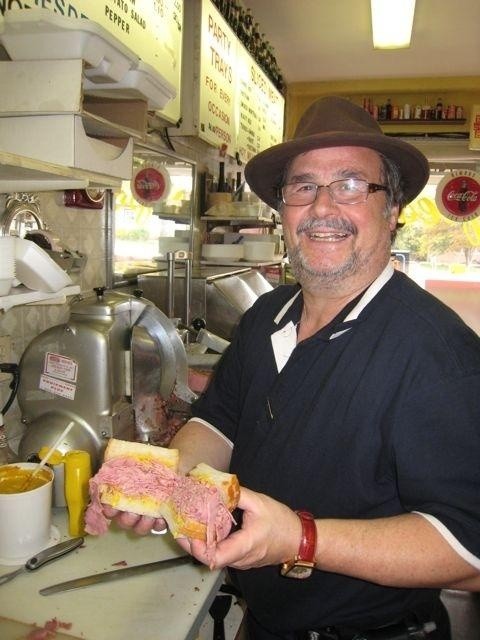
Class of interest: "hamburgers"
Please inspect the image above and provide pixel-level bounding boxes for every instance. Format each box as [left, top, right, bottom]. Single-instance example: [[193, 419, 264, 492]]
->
[[82, 436, 181, 537], [158, 463, 241, 571]]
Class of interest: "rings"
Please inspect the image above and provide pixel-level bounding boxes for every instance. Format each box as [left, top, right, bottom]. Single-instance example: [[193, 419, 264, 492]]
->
[[151, 528, 170, 535]]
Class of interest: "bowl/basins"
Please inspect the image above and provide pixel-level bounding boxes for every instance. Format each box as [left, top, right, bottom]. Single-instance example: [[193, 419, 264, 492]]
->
[[198, 240, 275, 264]]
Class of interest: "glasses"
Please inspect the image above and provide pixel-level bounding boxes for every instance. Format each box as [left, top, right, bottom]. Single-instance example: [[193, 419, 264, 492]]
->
[[275, 178, 391, 205]]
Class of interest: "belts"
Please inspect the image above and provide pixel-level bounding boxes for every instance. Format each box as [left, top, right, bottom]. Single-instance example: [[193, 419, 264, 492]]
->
[[245, 598, 440, 640]]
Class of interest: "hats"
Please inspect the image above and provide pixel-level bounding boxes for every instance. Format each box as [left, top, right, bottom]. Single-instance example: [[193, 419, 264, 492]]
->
[[244, 96, 430, 212]]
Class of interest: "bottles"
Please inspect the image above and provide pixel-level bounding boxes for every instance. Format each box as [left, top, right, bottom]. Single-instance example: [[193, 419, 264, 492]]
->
[[208, 162, 242, 207], [363, 95, 463, 120], [457, 179, 468, 212], [63, 448, 91, 536], [217, 0, 284, 86]]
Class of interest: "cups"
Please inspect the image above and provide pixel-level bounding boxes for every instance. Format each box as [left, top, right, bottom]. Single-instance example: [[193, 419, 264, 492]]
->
[[0, 463, 55, 557]]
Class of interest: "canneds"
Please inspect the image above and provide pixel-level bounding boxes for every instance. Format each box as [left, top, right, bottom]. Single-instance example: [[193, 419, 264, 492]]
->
[[359, 98, 463, 121]]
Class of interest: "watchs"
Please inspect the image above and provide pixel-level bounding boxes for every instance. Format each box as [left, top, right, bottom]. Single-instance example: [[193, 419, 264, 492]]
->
[[281, 509, 318, 580]]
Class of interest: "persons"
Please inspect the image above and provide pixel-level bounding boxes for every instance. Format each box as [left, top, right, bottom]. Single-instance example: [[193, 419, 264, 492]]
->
[[101, 95, 480, 640]]
